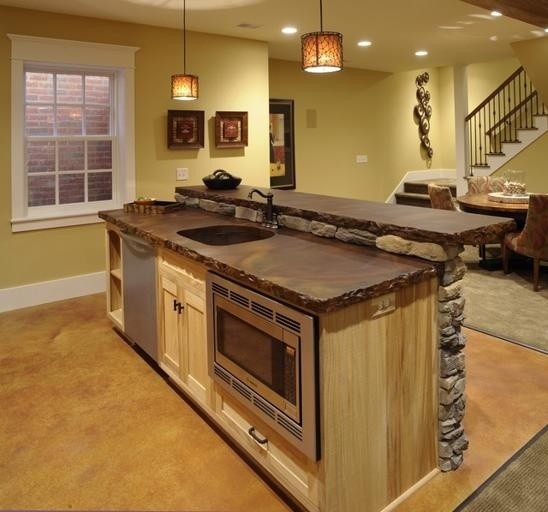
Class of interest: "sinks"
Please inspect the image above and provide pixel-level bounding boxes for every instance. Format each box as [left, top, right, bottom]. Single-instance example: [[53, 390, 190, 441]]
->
[[176, 224, 275, 246]]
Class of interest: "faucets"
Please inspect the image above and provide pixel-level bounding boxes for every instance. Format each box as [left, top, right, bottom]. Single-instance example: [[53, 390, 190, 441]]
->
[[247, 188, 274, 225]]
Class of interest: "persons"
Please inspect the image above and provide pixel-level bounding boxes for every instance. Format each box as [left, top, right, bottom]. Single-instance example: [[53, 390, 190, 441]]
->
[[269, 124, 275, 163]]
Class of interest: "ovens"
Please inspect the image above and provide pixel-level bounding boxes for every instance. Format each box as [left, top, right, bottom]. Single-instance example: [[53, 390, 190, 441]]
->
[[117, 231, 159, 360]]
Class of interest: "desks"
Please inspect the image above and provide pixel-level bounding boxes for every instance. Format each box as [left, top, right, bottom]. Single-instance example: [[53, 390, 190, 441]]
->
[[454, 189, 547, 274]]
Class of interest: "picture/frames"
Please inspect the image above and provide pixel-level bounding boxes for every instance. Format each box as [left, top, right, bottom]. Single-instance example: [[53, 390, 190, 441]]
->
[[270, 98, 298, 190], [162, 108, 209, 153], [212, 109, 251, 151]]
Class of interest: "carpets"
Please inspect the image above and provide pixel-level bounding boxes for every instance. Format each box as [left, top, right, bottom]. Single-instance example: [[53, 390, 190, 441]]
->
[[452, 425, 548, 511], [459, 242, 548, 355]]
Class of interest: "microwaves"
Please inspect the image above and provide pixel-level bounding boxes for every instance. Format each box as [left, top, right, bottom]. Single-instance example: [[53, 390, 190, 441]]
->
[[200, 272, 321, 462]]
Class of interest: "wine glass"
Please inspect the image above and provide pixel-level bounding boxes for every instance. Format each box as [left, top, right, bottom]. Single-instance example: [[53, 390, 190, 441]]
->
[[503, 170, 526, 197]]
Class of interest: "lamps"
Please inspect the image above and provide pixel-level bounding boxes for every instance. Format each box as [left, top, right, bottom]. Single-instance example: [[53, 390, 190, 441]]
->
[[296, 2, 344, 78], [165, 0, 203, 103]]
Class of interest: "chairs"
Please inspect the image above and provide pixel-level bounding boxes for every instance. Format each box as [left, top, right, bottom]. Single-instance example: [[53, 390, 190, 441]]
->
[[424, 180, 486, 270], [460, 176, 510, 196], [498, 192, 546, 293]]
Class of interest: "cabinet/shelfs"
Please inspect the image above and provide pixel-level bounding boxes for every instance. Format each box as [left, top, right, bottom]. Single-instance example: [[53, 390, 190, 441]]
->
[[104, 227, 123, 333], [155, 248, 212, 417]]
